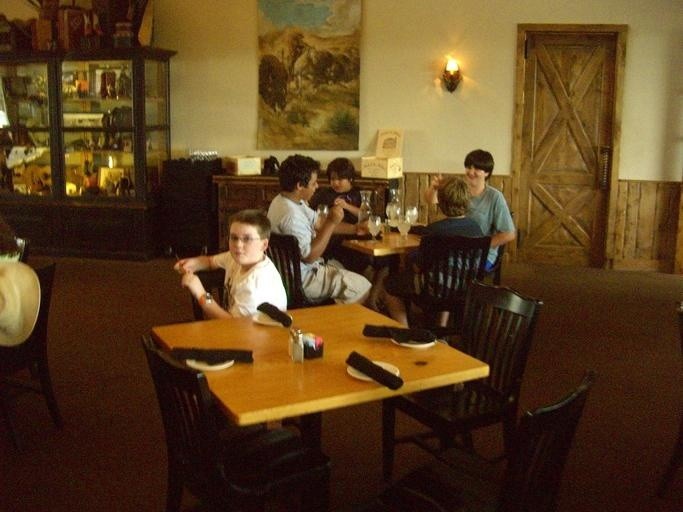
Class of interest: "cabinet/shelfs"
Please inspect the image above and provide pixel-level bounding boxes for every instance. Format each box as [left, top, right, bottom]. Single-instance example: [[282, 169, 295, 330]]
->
[[0, 45, 178, 263], [212, 176, 399, 253]]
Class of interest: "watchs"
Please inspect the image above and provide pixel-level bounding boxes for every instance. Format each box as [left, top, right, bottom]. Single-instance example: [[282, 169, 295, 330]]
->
[[197, 292, 212, 306]]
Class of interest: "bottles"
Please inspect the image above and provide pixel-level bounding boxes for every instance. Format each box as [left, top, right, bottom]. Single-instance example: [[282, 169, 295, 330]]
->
[[290, 329, 305, 363], [357, 189, 372, 226]]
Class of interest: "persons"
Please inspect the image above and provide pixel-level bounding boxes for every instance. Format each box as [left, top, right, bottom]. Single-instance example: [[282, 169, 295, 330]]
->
[[378, 174, 486, 342], [265, 153, 375, 307], [173, 209, 288, 321], [307, 158, 370, 276], [421, 149, 518, 281]]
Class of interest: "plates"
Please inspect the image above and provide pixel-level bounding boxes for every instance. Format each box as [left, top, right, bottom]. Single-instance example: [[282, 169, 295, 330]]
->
[[251, 312, 294, 327], [184, 355, 235, 371], [392, 335, 437, 349], [345, 358, 400, 383]]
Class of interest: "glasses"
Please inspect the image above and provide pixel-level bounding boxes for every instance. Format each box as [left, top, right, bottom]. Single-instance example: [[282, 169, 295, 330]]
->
[[226, 235, 260, 244]]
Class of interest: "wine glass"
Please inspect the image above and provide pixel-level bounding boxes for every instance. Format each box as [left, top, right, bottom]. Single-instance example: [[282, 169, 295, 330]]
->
[[397, 214, 411, 242], [367, 215, 382, 244]]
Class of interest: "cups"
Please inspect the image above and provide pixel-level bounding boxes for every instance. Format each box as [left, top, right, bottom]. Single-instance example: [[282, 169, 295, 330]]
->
[[316, 204, 330, 219], [406, 206, 419, 225]]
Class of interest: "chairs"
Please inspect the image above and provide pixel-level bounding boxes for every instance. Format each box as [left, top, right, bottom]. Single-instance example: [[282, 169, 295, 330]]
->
[[0, 237, 65, 452]]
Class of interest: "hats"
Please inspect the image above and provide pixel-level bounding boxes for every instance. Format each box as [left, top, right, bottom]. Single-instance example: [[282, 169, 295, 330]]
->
[[0, 260, 40, 348]]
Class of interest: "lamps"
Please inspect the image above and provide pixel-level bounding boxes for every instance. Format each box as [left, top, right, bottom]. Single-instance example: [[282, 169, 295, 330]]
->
[[443, 59, 462, 93]]
